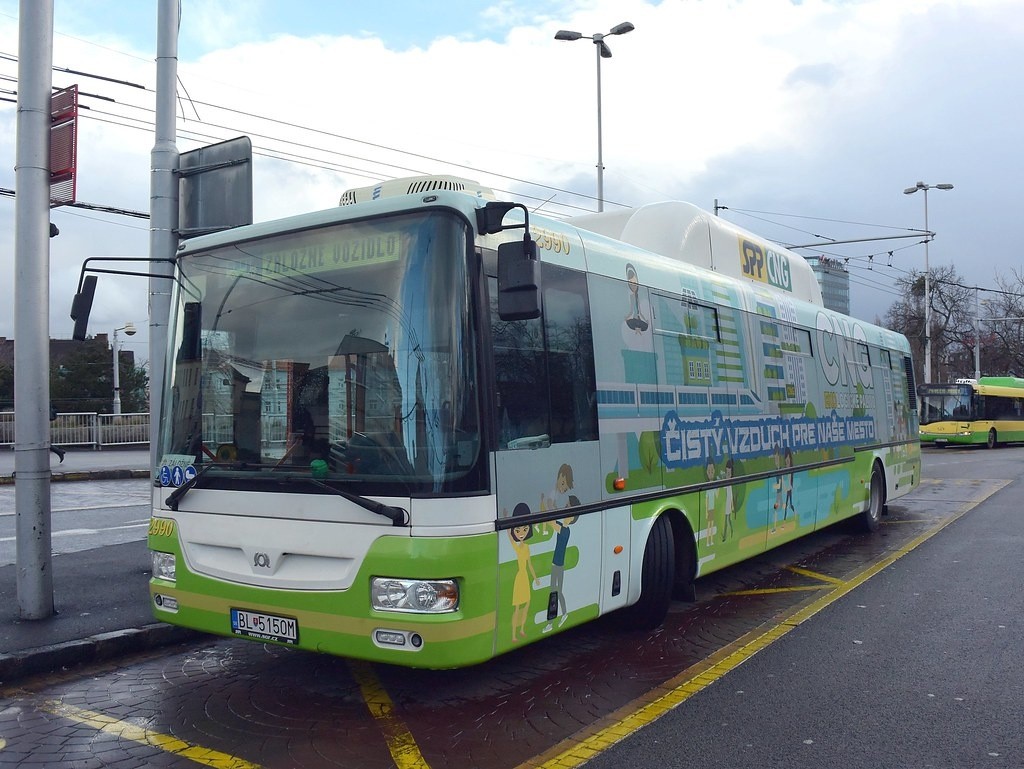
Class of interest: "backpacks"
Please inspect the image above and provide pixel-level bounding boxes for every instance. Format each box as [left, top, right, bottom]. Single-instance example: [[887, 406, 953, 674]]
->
[[50, 404, 57, 420]]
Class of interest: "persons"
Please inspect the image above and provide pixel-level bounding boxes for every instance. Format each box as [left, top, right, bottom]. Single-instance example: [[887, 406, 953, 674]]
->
[[493, 379, 548, 449], [50, 444, 65, 463]]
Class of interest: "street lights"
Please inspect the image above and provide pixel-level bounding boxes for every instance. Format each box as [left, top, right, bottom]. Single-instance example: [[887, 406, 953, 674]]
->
[[554, 22, 636, 212], [903, 181, 955, 423], [111, 320, 137, 425]]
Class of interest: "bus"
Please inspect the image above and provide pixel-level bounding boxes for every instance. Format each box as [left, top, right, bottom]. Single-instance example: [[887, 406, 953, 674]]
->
[[916, 376, 1024, 450], [69, 172, 923, 670]]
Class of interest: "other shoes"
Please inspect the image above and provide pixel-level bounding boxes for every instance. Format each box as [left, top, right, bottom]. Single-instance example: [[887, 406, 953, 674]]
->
[[60, 450, 65, 463]]
